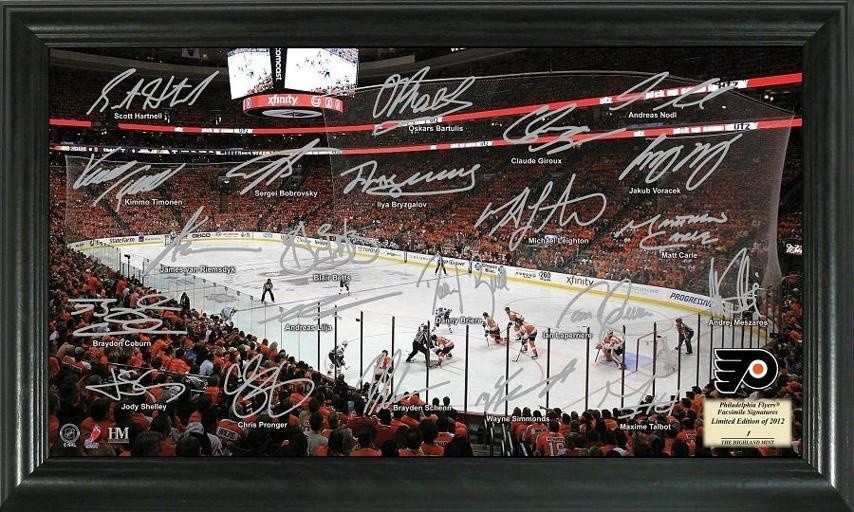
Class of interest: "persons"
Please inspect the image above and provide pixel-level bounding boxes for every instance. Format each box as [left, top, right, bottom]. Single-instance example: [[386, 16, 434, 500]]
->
[[41, 46, 808, 460]]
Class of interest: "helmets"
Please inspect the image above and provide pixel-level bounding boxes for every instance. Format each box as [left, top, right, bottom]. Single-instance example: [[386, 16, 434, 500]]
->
[[341, 339, 389, 358], [419, 305, 522, 344], [607, 317, 683, 337]]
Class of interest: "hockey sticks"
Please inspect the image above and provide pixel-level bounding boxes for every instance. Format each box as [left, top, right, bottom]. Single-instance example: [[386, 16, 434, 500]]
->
[[592, 349, 601, 366], [483, 325, 490, 348], [513, 341, 524, 362], [343, 364, 351, 370]]
